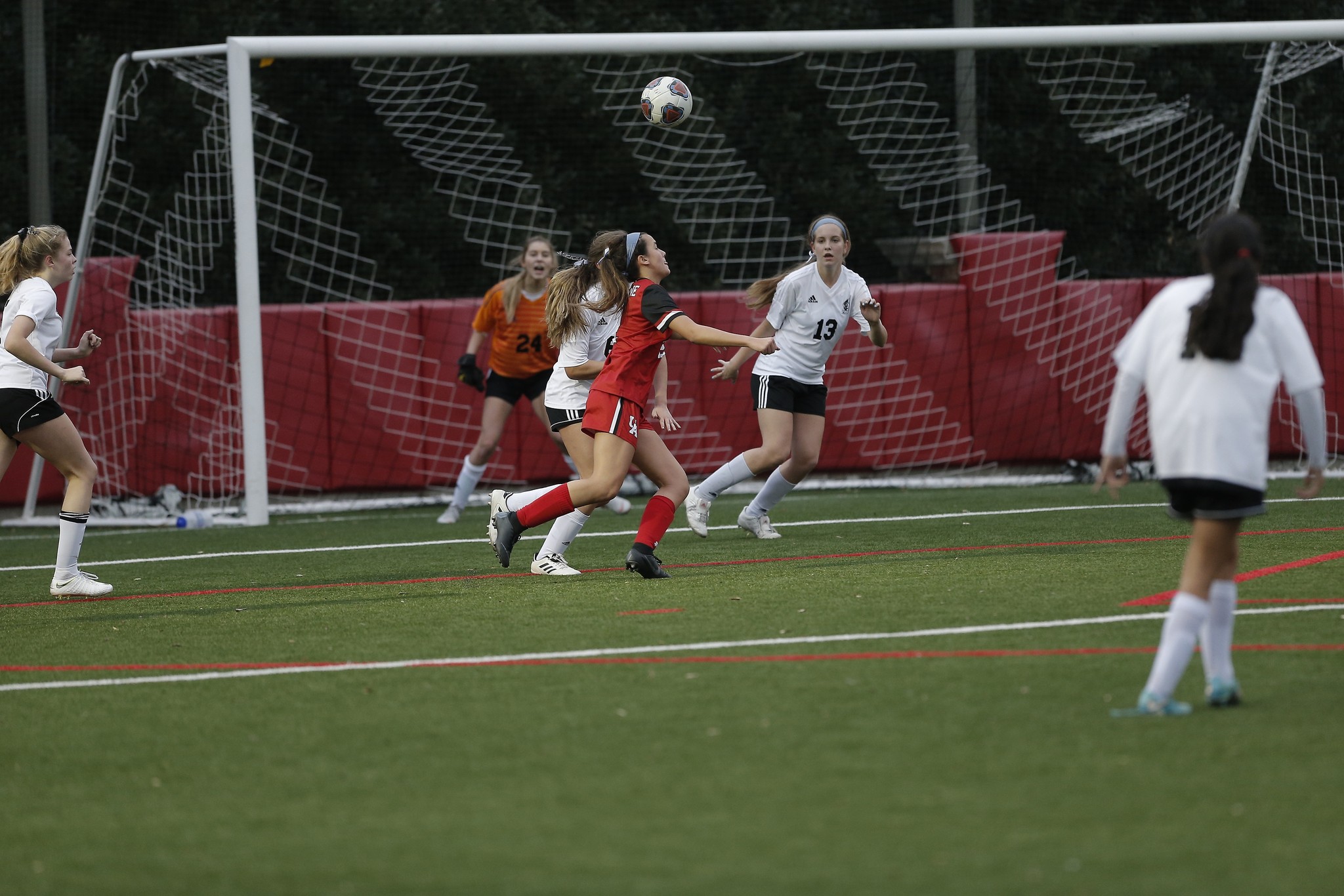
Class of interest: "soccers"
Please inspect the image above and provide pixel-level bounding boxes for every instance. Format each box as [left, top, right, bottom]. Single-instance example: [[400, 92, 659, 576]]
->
[[642, 75, 695, 128]]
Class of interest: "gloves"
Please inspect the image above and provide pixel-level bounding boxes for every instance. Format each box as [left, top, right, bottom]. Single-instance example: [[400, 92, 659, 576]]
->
[[457, 354, 486, 392]]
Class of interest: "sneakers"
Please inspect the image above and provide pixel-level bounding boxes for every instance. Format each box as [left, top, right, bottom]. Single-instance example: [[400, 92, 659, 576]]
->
[[625, 548, 672, 579], [487, 489, 511, 551], [437, 505, 467, 524], [683, 485, 711, 538], [49, 573, 113, 599], [600, 496, 632, 515], [530, 552, 582, 576], [1201, 679, 1241, 705], [736, 506, 781, 540], [1136, 690, 1191, 715], [490, 512, 519, 568]]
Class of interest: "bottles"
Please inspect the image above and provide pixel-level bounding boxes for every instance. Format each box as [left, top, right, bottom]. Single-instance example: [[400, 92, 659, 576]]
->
[[176, 508, 214, 529]]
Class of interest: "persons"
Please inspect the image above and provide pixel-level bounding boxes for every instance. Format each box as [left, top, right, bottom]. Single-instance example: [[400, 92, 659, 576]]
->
[[487, 229, 681, 576], [1095, 210, 1330, 719], [491, 232, 782, 571], [436, 235, 632, 525], [0, 223, 121, 598], [682, 214, 890, 538]]
